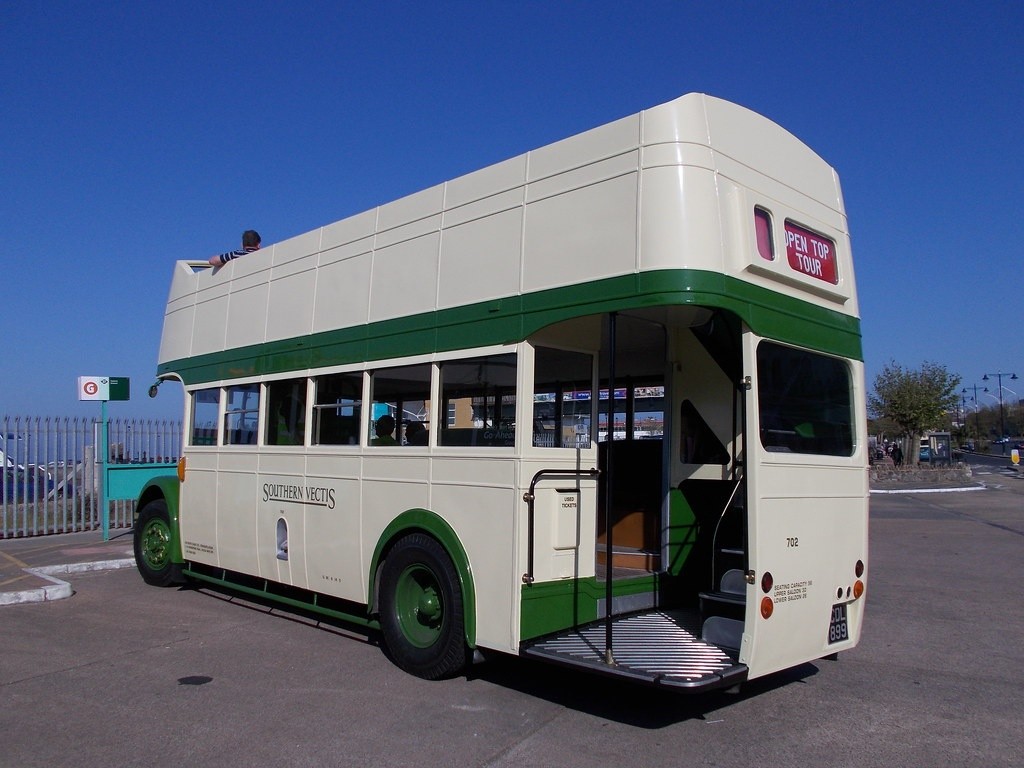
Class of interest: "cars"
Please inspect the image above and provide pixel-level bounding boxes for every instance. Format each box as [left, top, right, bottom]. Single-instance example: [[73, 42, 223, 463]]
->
[[919, 446, 930, 462], [960, 441, 975, 451], [1015, 441, 1024, 449], [993, 436, 1010, 445], [0, 466, 79, 503]]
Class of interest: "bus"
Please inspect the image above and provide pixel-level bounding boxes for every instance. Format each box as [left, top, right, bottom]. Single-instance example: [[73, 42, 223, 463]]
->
[[132, 89, 872, 689]]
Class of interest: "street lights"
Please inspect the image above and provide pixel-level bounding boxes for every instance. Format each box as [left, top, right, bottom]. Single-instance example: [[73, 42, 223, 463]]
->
[[957, 395, 974, 443], [982, 370, 1019, 458], [961, 383, 989, 452]]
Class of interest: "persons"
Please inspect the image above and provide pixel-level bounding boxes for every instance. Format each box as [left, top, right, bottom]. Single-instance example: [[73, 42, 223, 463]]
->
[[404, 422, 426, 442], [891, 445, 903, 468], [276, 378, 307, 446], [968, 441, 973, 454], [881, 444, 889, 456], [372, 415, 402, 445], [209, 230, 261, 266]]
